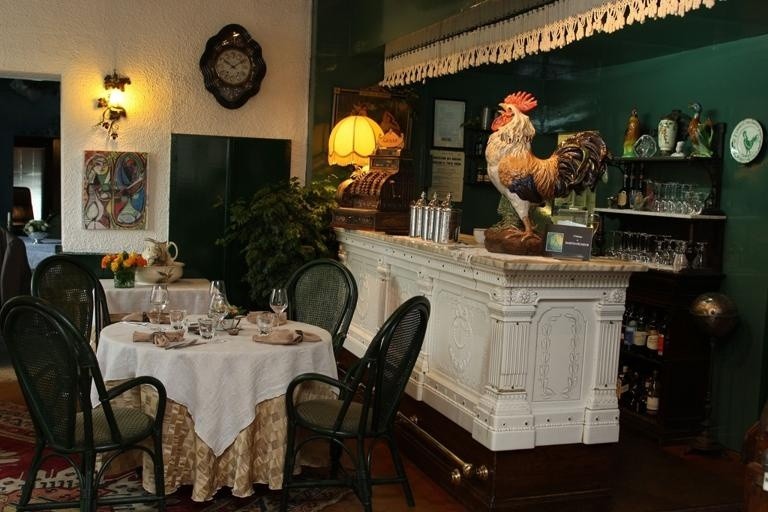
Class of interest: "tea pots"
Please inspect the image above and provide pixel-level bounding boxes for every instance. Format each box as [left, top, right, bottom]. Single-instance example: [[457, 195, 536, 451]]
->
[[139, 238, 179, 266]]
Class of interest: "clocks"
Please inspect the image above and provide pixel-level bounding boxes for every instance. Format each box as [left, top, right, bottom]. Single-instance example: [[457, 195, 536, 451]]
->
[[198, 24, 266, 110]]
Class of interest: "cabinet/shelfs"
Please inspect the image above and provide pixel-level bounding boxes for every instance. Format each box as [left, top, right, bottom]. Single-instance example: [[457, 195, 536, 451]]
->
[[590, 121, 727, 443], [463, 121, 495, 189]]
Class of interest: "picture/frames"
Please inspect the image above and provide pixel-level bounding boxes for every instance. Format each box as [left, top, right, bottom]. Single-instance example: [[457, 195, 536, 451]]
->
[[328, 86, 415, 163]]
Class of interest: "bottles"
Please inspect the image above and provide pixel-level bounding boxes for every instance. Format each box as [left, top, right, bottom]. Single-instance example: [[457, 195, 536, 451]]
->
[[613, 161, 648, 209], [613, 365, 661, 419], [616, 302, 666, 356]]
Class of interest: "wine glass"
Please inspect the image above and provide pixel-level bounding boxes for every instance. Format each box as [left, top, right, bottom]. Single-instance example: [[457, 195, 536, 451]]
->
[[268, 288, 289, 327], [206, 294, 227, 333], [650, 182, 702, 215], [604, 228, 709, 273], [207, 280, 226, 300], [166, 309, 186, 331], [147, 284, 169, 331]]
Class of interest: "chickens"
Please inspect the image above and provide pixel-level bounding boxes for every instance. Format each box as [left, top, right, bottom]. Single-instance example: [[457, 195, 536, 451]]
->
[[484, 89, 610, 242]]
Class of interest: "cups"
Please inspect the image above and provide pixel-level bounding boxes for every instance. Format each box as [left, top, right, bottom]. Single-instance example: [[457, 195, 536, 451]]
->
[[197, 318, 215, 339], [256, 313, 274, 336]]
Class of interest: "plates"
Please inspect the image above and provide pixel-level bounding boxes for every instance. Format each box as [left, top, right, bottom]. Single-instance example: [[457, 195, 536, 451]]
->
[[726, 116, 764, 166]]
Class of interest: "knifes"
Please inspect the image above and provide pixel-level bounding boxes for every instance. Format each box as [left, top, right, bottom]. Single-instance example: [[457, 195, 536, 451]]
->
[[165, 338, 206, 349]]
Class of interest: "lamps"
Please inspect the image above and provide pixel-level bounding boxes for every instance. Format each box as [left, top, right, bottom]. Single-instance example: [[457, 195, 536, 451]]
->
[[326, 112, 387, 208], [92, 69, 132, 141]]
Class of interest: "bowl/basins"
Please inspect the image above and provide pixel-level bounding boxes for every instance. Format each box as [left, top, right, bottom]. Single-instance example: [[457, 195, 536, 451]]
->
[[134, 261, 184, 284]]
